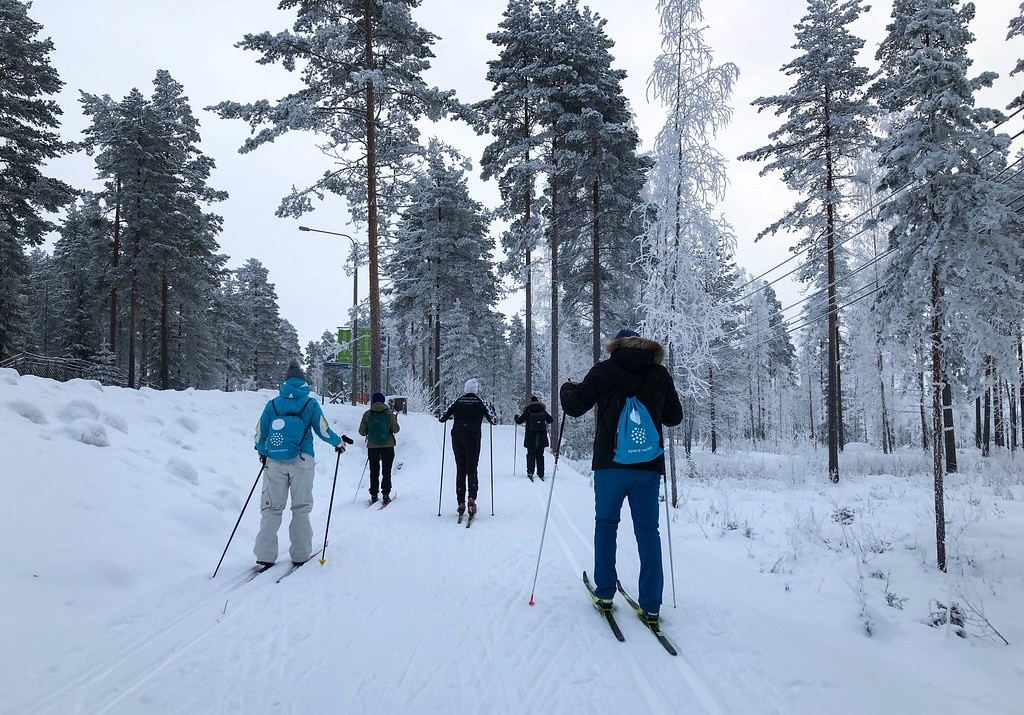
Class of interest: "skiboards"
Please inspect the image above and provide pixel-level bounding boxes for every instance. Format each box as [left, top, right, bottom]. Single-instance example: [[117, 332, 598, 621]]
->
[[246, 539, 330, 584], [582, 569, 677, 657], [456, 495, 478, 529], [526, 469, 545, 483], [364, 490, 397, 510]]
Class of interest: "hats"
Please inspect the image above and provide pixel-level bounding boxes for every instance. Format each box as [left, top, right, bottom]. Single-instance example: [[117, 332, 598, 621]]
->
[[284, 356, 304, 380], [372, 392, 385, 403], [464, 378, 478, 394], [615, 330, 641, 338]]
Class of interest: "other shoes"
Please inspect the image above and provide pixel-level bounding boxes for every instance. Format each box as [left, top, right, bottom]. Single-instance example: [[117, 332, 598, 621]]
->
[[256, 559, 274, 566], [294, 560, 304, 566]]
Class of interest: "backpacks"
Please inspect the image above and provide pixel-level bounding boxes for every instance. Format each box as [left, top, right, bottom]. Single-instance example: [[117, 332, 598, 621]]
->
[[264, 398, 313, 460], [528, 412, 546, 431], [613, 396, 665, 464], [366, 410, 389, 444]]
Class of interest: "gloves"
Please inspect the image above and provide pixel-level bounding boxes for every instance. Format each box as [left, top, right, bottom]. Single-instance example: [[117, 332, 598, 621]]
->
[[335, 438, 346, 454], [514, 414, 518, 419]]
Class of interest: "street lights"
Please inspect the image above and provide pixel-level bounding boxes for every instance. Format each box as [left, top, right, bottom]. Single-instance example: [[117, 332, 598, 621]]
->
[[299, 226, 357, 407]]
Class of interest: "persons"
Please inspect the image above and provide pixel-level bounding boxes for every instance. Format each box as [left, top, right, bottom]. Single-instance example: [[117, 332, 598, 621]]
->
[[559, 331, 684, 624], [438, 377, 498, 514], [254, 359, 346, 565], [514, 396, 554, 479], [358, 393, 400, 505]]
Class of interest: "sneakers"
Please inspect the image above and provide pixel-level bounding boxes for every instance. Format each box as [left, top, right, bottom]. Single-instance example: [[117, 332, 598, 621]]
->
[[638, 605, 659, 622], [371, 494, 378, 503], [467, 497, 476, 514], [383, 494, 391, 504], [526, 471, 534, 476], [457, 503, 465, 512], [593, 596, 613, 611], [537, 471, 544, 477]]
